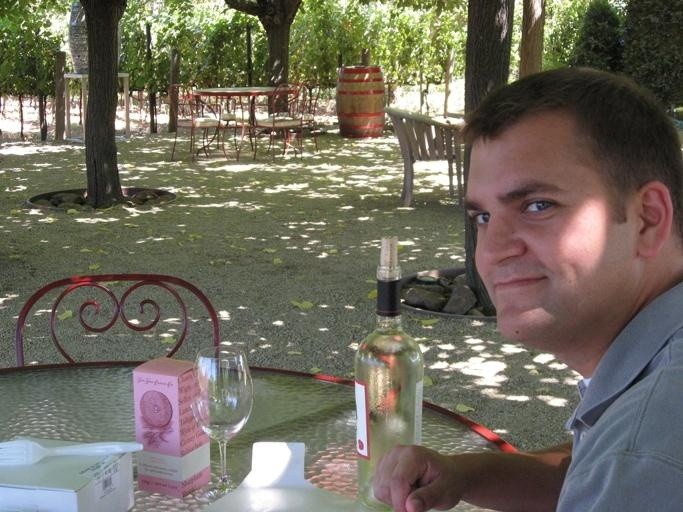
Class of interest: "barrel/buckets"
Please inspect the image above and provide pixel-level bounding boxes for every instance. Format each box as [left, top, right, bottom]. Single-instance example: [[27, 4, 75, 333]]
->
[[334, 65, 385, 139]]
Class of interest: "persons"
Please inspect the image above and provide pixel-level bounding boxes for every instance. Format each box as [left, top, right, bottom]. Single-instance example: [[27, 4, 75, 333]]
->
[[374, 67, 681, 512]]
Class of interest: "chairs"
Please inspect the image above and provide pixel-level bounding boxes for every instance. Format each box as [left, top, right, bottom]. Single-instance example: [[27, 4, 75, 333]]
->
[[384, 108, 461, 206], [248, 90, 276, 160], [217, 97, 249, 161], [14, 273, 218, 367], [281, 79, 319, 160], [268, 82, 310, 162], [169, 83, 217, 159]]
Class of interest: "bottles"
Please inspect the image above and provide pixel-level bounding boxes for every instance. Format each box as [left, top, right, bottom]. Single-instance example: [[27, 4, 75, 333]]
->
[[355, 237, 423, 509]]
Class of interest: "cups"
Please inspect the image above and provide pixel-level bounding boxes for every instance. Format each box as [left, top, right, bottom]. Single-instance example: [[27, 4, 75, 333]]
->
[[189, 344, 254, 502]]
